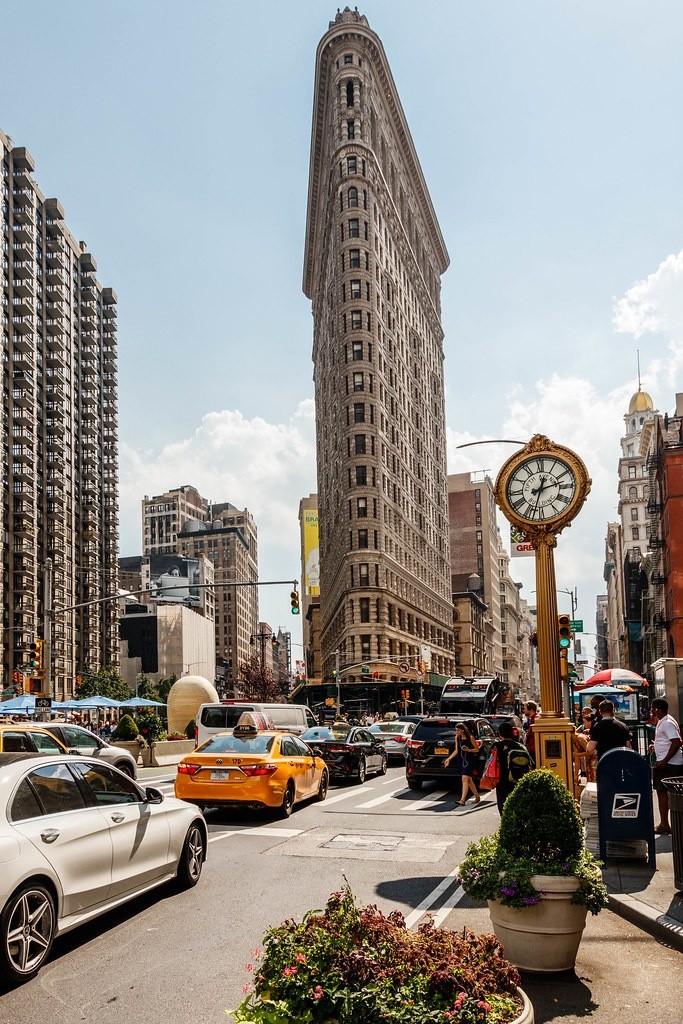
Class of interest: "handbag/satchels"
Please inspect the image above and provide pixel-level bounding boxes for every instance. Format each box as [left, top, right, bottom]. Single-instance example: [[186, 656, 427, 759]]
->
[[479, 747, 501, 789]]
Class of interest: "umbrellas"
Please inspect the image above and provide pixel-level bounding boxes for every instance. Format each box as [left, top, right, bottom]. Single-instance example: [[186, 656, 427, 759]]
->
[[584, 668, 646, 687], [123, 698, 167, 714], [0, 695, 134, 735]]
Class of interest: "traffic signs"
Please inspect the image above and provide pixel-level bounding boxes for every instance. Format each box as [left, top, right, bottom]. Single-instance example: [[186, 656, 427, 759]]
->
[[570, 619, 584, 632]]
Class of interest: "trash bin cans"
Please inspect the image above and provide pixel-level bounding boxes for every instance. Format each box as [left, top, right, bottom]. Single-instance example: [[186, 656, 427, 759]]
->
[[661, 776, 683, 891], [596, 747, 656, 870]]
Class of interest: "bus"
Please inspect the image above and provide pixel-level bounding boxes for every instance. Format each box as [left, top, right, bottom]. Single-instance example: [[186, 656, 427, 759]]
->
[[438, 675, 526, 722]]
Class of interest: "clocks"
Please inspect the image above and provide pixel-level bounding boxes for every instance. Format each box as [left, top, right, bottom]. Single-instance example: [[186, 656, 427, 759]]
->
[[491, 433, 594, 543]]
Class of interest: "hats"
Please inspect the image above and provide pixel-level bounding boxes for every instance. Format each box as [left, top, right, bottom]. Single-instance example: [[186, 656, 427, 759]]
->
[[594, 694, 605, 702], [498, 724, 513, 738]]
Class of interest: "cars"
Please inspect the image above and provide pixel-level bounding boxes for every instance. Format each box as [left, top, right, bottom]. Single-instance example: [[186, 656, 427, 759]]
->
[[174, 711, 329, 819], [366, 720, 417, 759], [391, 714, 431, 725], [0, 755, 208, 982], [298, 723, 388, 785]]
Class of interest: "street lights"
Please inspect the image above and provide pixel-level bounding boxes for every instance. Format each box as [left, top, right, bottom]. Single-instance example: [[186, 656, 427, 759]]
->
[[170, 660, 207, 676], [135, 672, 143, 721], [418, 637, 445, 716], [279, 642, 309, 707], [530, 587, 577, 665], [568, 671, 580, 725], [582, 631, 621, 669], [249, 630, 277, 703]]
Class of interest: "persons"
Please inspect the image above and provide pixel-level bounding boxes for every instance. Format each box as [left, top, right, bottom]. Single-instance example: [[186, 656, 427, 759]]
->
[[575, 695, 630, 760], [445, 723, 480, 806], [68, 714, 118, 742], [522, 701, 542, 762], [343, 712, 385, 726], [12, 714, 20, 722], [156, 565, 181, 587], [487, 723, 535, 816], [21, 715, 29, 722], [649, 699, 683, 834]]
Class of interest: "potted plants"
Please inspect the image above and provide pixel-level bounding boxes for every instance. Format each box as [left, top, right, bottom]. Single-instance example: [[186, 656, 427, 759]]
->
[[109, 714, 142, 764], [228, 892, 535, 1024], [137, 716, 163, 766], [455, 767, 609, 972]]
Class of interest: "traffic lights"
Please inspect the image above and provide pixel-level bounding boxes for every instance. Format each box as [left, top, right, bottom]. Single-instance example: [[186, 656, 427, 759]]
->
[[417, 660, 421, 671], [558, 613, 571, 648], [290, 591, 301, 614], [401, 690, 406, 700], [138, 707, 144, 712], [29, 641, 43, 670], [15, 687, 25, 694], [76, 675, 82, 687], [399, 702, 404, 708], [421, 659, 429, 673], [17, 674, 23, 683], [406, 690, 410, 699], [12, 672, 17, 683], [372, 671, 380, 681], [326, 685, 338, 697]]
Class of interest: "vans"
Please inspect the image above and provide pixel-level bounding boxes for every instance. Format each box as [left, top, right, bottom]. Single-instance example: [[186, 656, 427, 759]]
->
[[194, 698, 320, 750]]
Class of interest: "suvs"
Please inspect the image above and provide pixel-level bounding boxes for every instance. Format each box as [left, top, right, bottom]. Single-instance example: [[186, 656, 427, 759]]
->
[[404, 712, 498, 791], [0, 718, 107, 795], [482, 713, 527, 746], [15, 721, 138, 782]]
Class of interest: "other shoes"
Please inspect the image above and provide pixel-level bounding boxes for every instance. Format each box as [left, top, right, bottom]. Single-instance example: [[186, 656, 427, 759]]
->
[[654, 823, 671, 835], [455, 800, 465, 806], [471, 798, 480, 804]]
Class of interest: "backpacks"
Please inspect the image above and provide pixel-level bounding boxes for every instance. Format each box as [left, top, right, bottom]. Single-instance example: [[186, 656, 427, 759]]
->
[[503, 740, 529, 784]]
